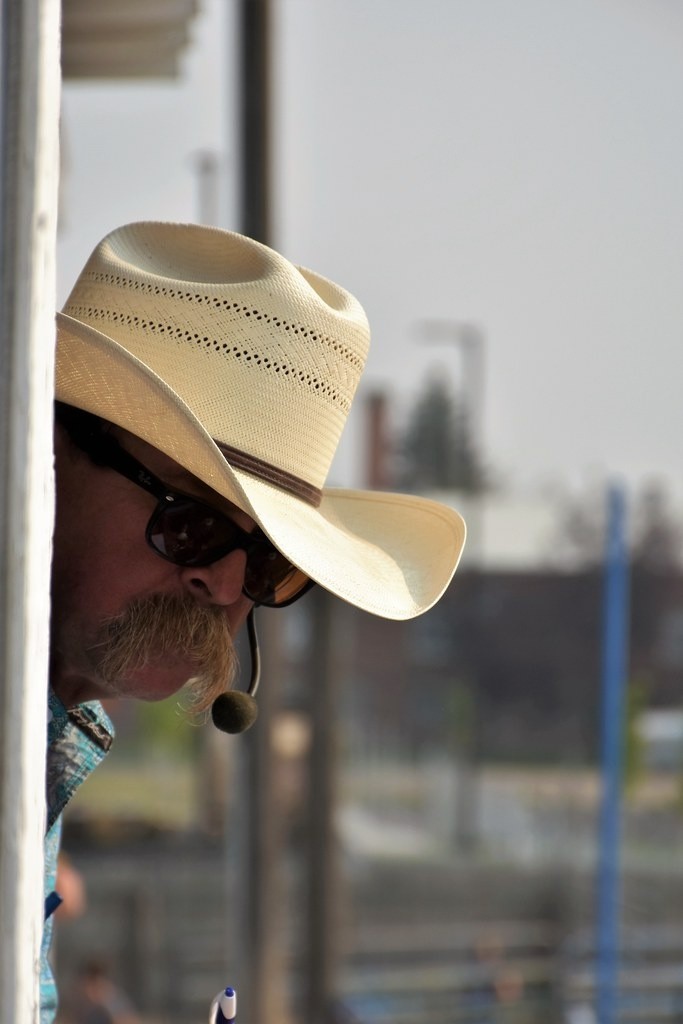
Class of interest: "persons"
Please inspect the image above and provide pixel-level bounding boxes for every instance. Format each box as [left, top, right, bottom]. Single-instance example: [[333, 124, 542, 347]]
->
[[39, 221, 467, 1024]]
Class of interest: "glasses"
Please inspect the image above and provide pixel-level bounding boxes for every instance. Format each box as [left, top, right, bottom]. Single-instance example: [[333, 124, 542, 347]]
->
[[51, 399, 318, 609]]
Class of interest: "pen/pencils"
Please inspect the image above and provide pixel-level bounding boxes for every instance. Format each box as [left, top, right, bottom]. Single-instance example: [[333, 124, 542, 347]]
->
[[208, 988, 239, 1024]]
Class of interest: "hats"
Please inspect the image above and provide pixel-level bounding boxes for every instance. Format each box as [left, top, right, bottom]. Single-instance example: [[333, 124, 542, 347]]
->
[[55, 221, 467, 619]]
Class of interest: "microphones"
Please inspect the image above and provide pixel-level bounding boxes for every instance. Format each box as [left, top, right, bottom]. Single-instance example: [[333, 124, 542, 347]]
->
[[211, 606, 260, 735]]
